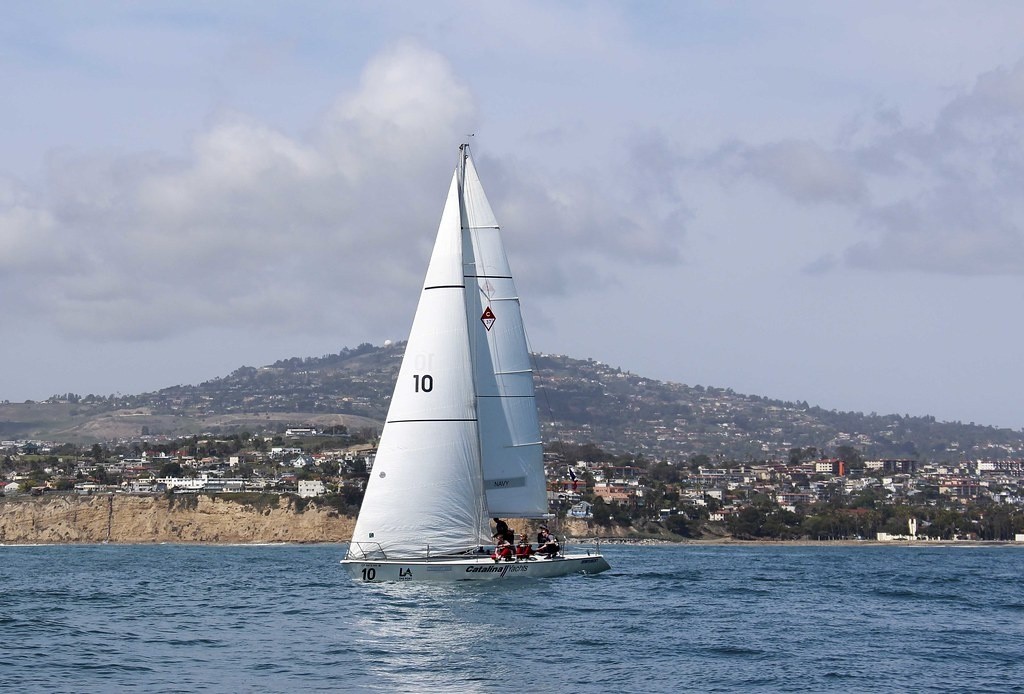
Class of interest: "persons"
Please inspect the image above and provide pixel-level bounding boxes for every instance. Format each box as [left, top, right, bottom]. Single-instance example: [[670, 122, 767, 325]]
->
[[536, 525, 560, 559], [486, 550, 491, 555], [479, 546, 484, 553], [493, 517, 532, 560]]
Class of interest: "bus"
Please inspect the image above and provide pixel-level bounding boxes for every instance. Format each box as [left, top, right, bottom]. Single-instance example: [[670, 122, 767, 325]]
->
[[659, 509, 678, 520], [31, 487, 50, 496]]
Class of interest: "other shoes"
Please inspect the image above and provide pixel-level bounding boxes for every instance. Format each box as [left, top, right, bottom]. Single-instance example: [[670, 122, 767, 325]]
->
[[544, 556, 551, 559], [508, 558, 515, 561], [517, 557, 521, 561]]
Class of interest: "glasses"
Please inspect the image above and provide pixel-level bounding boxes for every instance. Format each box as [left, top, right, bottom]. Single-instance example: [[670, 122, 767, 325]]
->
[[541, 531, 546, 533]]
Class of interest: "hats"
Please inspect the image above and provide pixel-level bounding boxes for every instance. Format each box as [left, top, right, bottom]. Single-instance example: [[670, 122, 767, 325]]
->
[[540, 525, 547, 529]]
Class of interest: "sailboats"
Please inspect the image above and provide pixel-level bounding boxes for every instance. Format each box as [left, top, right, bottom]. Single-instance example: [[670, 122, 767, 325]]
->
[[341, 143, 613, 583]]
